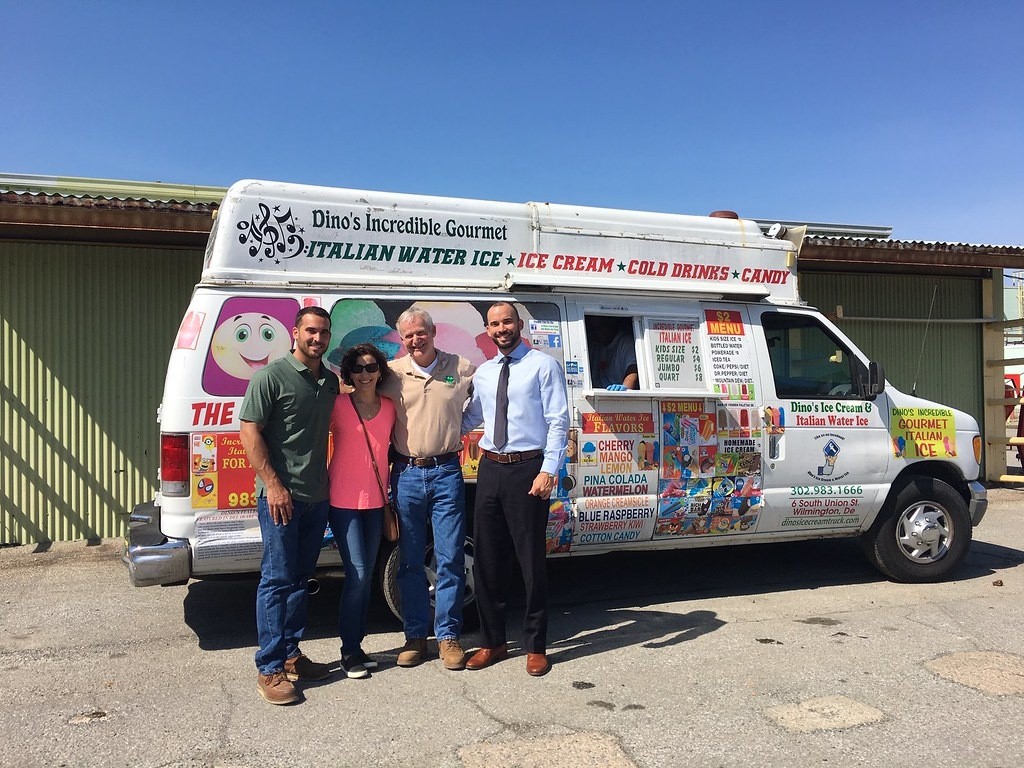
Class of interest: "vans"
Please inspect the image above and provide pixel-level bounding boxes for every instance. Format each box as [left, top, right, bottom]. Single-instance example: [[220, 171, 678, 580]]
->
[[124, 180, 989, 627]]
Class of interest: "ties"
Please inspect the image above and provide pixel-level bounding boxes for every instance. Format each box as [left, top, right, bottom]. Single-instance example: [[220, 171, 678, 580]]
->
[[493, 357, 512, 450]]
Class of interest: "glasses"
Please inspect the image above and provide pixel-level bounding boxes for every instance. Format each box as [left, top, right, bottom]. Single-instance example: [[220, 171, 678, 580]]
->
[[350, 363, 380, 373]]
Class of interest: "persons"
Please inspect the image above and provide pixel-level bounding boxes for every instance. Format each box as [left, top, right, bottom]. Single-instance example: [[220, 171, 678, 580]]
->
[[460, 302, 570, 675], [341, 307, 478, 670], [238, 306, 340, 706], [587, 315, 638, 391], [325, 344, 397, 680]]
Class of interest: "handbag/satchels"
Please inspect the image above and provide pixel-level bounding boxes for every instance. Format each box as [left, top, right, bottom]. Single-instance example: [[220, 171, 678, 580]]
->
[[382, 504, 399, 542]]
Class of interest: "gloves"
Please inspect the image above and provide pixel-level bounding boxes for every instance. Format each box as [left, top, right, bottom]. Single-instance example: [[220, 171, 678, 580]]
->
[[606, 384, 626, 391]]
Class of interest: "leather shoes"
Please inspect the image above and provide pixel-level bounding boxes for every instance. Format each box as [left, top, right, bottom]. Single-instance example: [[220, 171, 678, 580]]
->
[[466, 643, 508, 669], [526, 653, 549, 676]]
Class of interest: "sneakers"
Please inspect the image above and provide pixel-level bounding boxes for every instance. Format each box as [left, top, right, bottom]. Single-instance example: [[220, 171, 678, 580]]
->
[[257, 671, 299, 704], [284, 654, 331, 681], [437, 638, 465, 670], [397, 638, 428, 666]]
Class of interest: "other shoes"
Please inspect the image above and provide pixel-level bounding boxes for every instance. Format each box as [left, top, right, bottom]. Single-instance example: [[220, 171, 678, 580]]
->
[[359, 648, 378, 668], [340, 654, 368, 678]]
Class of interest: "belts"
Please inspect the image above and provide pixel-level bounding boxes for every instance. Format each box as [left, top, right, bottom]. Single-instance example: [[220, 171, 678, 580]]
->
[[484, 450, 542, 463], [396, 453, 457, 467]]
